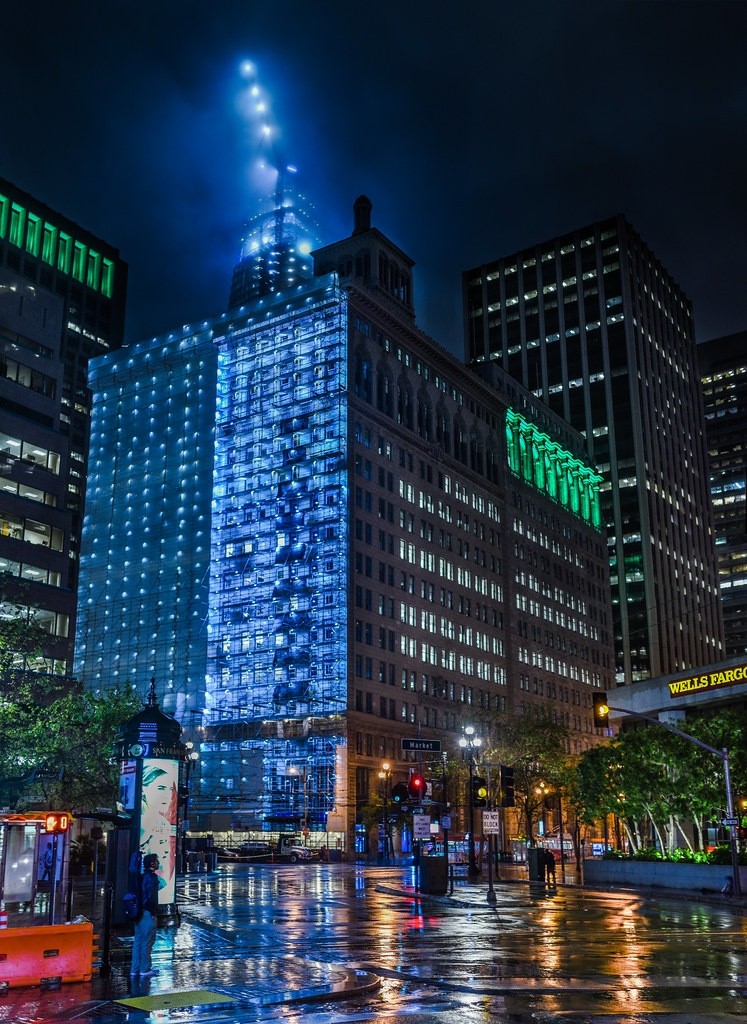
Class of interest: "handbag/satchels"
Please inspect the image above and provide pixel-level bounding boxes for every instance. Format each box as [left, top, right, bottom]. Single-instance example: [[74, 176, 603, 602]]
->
[[122, 894, 141, 922]]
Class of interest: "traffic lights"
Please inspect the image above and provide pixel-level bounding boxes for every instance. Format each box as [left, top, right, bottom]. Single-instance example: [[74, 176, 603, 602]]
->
[[392, 783, 409, 802], [409, 778, 427, 796], [592, 692, 609, 727], [473, 776, 487, 807], [46, 813, 68, 834], [500, 766, 515, 807]]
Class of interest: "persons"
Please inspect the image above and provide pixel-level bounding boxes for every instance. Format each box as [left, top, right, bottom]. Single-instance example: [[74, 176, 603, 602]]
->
[[24, 901, 30, 912], [320, 847, 324, 863], [545, 849, 556, 880], [142, 765, 174, 863], [130, 854, 160, 977], [38, 843, 61, 880]]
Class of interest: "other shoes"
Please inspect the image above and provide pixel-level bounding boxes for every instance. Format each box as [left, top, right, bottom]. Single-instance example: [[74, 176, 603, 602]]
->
[[131, 968, 160, 976]]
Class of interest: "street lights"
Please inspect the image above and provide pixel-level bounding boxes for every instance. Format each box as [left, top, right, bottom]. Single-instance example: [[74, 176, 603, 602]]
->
[[536, 782, 550, 848], [289, 766, 310, 846], [379, 763, 393, 852], [181, 742, 199, 874], [459, 726, 481, 869]]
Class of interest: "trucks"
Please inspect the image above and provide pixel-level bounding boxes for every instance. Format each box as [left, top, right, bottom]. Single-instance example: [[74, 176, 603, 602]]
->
[[239, 837, 313, 863]]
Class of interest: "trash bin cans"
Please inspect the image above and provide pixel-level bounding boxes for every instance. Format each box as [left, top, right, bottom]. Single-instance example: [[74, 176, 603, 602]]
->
[[420, 853, 448, 895]]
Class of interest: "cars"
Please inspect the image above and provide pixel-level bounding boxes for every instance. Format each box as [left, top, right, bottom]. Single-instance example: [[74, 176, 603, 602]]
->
[[217, 847, 239, 861]]
